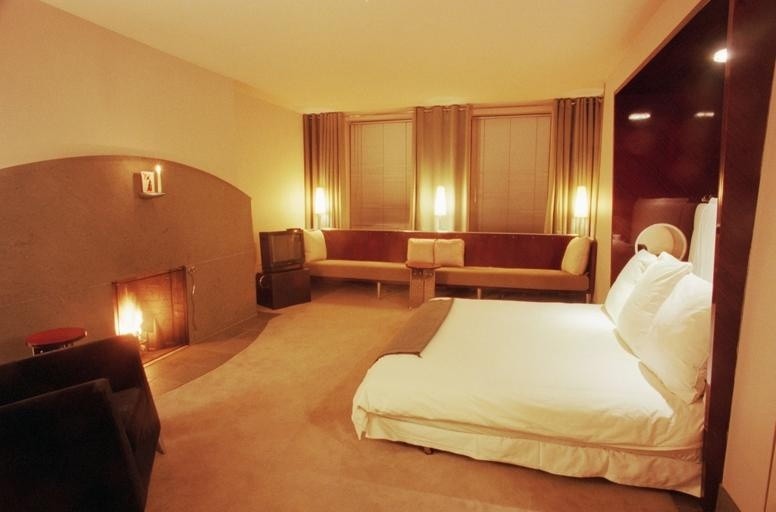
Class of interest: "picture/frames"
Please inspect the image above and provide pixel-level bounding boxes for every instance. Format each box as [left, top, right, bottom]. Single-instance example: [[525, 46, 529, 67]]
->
[[140, 171, 155, 193]]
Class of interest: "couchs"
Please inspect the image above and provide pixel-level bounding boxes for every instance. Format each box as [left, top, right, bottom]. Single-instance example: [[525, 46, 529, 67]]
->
[[305, 228, 438, 308], [424, 232, 597, 303]]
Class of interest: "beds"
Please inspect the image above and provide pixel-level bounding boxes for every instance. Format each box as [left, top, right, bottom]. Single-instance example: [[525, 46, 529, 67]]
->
[[351, 197, 718, 500]]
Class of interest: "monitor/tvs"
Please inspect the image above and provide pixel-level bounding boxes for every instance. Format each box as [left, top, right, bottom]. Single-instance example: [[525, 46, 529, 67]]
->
[[259, 229, 305, 273]]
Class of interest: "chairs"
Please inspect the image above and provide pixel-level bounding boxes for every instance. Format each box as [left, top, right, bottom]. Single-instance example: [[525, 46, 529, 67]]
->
[[0, 333, 162, 512]]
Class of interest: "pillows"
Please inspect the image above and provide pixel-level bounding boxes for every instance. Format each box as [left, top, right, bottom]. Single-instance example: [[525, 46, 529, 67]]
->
[[434, 238, 465, 267], [561, 237, 591, 276], [602, 248, 714, 405], [406, 237, 434, 265], [304, 230, 327, 262]]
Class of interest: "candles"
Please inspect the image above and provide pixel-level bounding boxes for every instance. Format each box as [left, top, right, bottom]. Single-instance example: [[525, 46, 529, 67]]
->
[[155, 165, 162, 193]]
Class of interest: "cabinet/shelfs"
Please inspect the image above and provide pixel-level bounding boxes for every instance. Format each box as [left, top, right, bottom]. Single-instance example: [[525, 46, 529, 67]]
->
[[255, 267, 311, 310]]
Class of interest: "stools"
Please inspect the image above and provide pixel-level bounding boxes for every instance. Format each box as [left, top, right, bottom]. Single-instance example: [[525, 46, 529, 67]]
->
[[23, 327, 88, 356]]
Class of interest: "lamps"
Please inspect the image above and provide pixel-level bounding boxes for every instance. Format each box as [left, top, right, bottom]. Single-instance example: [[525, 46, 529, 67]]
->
[[572, 186, 589, 236], [433, 185, 446, 230], [312, 187, 329, 230]]
